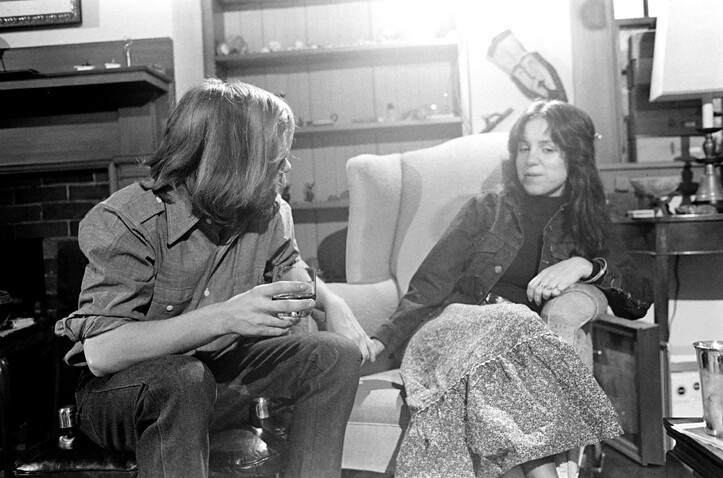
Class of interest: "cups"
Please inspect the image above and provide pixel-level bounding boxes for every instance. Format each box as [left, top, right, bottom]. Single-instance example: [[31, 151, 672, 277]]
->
[[692, 341, 723, 438], [272, 266, 318, 318]]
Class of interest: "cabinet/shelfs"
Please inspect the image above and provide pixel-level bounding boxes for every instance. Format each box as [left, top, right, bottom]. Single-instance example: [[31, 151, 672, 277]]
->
[[214, 37, 473, 281]]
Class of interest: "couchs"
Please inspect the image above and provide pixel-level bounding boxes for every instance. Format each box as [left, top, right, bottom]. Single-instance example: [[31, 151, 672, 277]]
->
[[313, 131, 607, 478]]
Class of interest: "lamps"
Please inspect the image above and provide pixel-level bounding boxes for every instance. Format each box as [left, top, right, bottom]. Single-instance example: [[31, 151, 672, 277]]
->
[[649, 0, 723, 200]]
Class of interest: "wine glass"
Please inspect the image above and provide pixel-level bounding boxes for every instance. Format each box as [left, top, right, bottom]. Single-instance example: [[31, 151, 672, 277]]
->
[[631, 176, 682, 215]]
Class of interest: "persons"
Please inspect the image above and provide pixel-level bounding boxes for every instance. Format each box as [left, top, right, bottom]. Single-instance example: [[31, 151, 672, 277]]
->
[[369, 100, 655, 478], [56, 76, 376, 478]]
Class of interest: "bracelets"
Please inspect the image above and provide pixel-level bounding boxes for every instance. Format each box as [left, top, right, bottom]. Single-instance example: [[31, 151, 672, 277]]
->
[[580, 257, 608, 284]]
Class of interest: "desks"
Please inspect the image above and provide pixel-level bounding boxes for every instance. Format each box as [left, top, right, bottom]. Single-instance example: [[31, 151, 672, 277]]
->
[[610, 211, 723, 460]]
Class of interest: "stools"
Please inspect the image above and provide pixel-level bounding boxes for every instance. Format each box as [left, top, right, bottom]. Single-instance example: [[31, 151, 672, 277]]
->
[[10, 407, 292, 478]]
